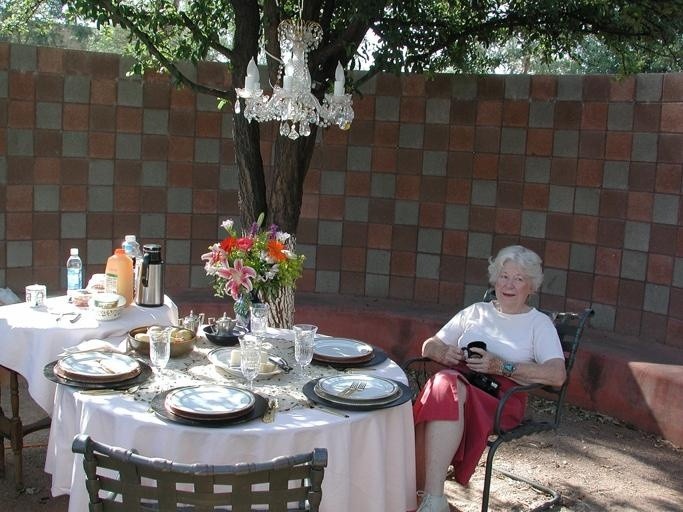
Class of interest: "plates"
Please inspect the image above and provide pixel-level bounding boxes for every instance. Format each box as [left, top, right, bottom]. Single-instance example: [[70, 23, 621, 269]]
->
[[44, 345, 293, 428], [303, 336, 413, 413]]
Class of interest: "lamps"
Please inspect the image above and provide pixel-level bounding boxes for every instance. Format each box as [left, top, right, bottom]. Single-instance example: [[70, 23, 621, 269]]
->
[[235, 1, 355, 141]]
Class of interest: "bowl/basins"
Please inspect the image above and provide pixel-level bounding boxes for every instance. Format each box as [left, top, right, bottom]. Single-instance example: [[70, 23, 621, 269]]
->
[[128, 322, 250, 357], [88, 292, 127, 321]]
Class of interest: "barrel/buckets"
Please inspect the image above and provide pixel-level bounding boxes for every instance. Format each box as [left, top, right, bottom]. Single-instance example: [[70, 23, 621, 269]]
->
[[105, 248, 134, 307]]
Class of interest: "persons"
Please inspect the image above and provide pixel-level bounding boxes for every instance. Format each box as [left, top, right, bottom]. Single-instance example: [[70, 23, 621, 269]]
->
[[413, 244, 567, 512]]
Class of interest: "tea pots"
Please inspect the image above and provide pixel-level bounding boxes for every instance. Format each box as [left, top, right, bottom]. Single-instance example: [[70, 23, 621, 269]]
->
[[179, 309, 233, 340]]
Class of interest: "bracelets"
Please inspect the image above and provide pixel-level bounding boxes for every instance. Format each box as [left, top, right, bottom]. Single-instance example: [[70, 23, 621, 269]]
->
[[497, 361, 514, 378]]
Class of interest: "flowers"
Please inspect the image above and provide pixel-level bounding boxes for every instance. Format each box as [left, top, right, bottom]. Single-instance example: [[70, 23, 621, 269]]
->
[[200, 210, 307, 316]]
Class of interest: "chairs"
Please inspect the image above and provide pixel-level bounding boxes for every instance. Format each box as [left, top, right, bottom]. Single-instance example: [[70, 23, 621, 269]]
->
[[72, 433, 328, 512], [400, 287, 595, 511]]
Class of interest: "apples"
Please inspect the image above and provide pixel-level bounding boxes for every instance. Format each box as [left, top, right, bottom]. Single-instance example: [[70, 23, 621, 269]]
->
[[134, 326, 192, 344]]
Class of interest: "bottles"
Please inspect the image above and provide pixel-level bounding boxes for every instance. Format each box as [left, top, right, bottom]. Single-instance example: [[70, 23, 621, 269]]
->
[[106, 235, 164, 306], [67, 248, 83, 298]]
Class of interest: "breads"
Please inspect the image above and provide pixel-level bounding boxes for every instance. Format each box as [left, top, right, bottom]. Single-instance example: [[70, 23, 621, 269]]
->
[[72, 290, 90, 307]]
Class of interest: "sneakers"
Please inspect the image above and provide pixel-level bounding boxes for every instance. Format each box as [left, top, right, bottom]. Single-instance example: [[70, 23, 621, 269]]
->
[[416, 490, 450, 512]]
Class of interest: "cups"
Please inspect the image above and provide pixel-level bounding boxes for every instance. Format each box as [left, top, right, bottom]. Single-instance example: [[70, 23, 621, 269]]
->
[[26, 284, 46, 307], [461, 341, 487, 358]]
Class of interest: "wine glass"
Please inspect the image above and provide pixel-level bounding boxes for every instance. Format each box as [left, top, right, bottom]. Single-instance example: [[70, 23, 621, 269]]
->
[[148, 330, 173, 386], [240, 303, 318, 389]]
